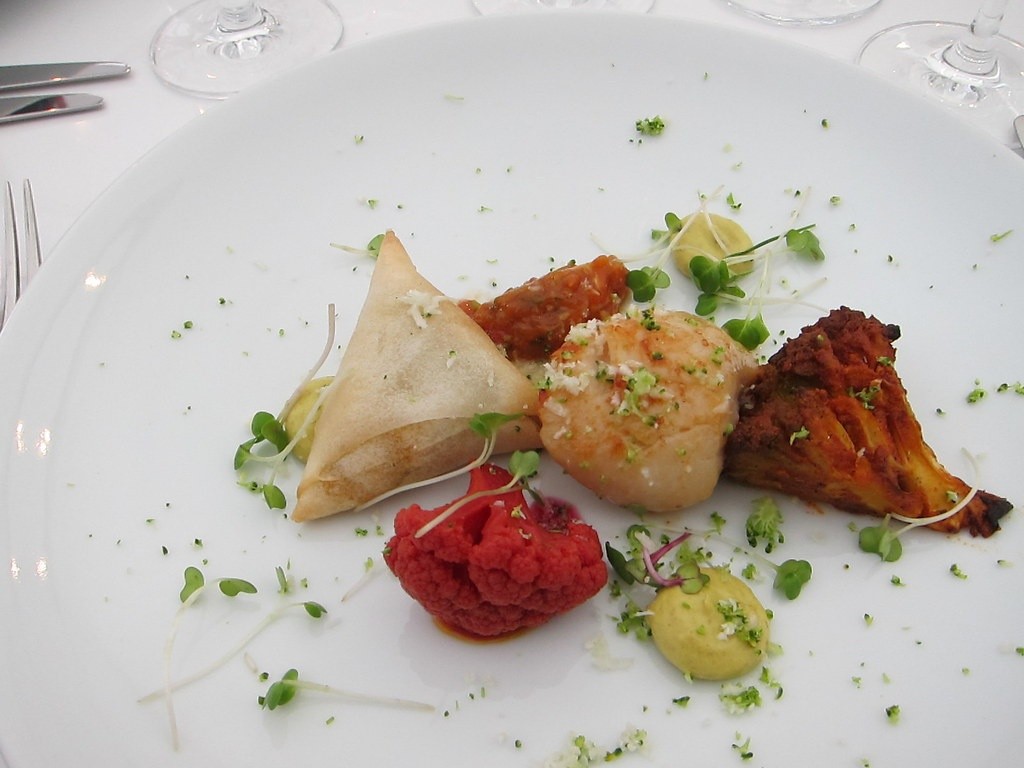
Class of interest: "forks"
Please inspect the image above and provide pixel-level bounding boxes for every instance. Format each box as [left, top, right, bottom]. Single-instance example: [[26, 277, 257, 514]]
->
[[0, 179, 41, 335]]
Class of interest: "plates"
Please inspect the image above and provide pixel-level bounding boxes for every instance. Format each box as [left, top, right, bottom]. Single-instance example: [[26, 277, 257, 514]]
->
[[0, 11, 1024, 768]]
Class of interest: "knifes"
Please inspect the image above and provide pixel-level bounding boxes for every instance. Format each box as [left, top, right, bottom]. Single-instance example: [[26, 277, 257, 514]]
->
[[0, 93, 104, 123], [0, 61, 131, 90], [1007, 113, 1024, 154]]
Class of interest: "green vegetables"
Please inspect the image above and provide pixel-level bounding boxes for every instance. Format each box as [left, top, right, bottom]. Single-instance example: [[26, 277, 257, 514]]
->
[[128, 191, 988, 714]]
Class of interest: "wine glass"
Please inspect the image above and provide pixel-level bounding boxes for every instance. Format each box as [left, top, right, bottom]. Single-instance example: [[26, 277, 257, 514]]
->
[[854, 0, 1024, 149], [149, 0, 344, 99]]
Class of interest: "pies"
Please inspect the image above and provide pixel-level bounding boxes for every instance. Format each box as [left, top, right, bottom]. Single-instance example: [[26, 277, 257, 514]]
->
[[290, 228, 546, 525]]
